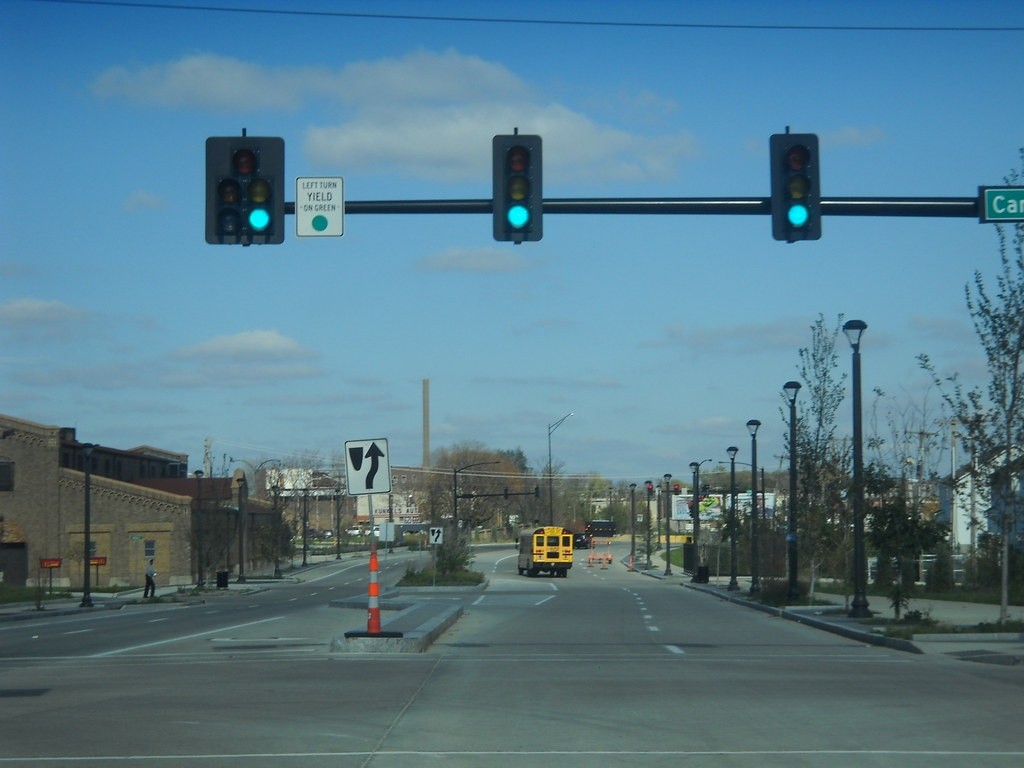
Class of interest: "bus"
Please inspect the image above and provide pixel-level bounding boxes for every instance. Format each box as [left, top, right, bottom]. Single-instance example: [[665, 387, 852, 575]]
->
[[515, 526, 574, 577]]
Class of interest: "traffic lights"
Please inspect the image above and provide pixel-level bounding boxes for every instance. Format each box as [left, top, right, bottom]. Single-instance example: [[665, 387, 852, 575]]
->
[[648, 484, 653, 495], [769, 133, 821, 240], [491, 134, 545, 241], [674, 485, 680, 495], [206, 135, 286, 245]]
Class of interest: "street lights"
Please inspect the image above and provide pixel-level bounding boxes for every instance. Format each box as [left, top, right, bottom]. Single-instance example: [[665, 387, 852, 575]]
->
[[782, 381, 802, 602], [663, 474, 673, 576], [548, 411, 575, 526], [746, 420, 762, 595], [335, 489, 341, 559], [78, 443, 95, 607], [644, 480, 652, 571], [842, 319, 873, 618], [272, 485, 281, 574], [718, 461, 767, 525], [689, 461, 699, 582], [453, 461, 500, 520], [236, 478, 246, 583], [302, 488, 308, 566], [194, 470, 204, 591], [726, 446, 740, 591], [629, 483, 637, 555]]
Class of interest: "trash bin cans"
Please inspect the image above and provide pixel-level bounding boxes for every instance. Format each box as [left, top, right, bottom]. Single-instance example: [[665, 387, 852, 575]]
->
[[694, 565, 710, 583], [216, 570, 229, 588]]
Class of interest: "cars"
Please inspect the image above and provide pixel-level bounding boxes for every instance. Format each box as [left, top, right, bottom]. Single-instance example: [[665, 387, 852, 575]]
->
[[573, 533, 591, 549], [344, 524, 379, 537]]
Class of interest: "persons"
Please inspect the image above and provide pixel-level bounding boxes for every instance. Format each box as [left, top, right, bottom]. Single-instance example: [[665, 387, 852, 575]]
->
[[142, 559, 158, 599]]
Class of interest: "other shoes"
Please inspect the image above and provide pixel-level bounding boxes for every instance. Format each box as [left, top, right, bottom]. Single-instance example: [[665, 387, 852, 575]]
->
[[143, 596, 149, 598], [150, 596, 157, 598]]
